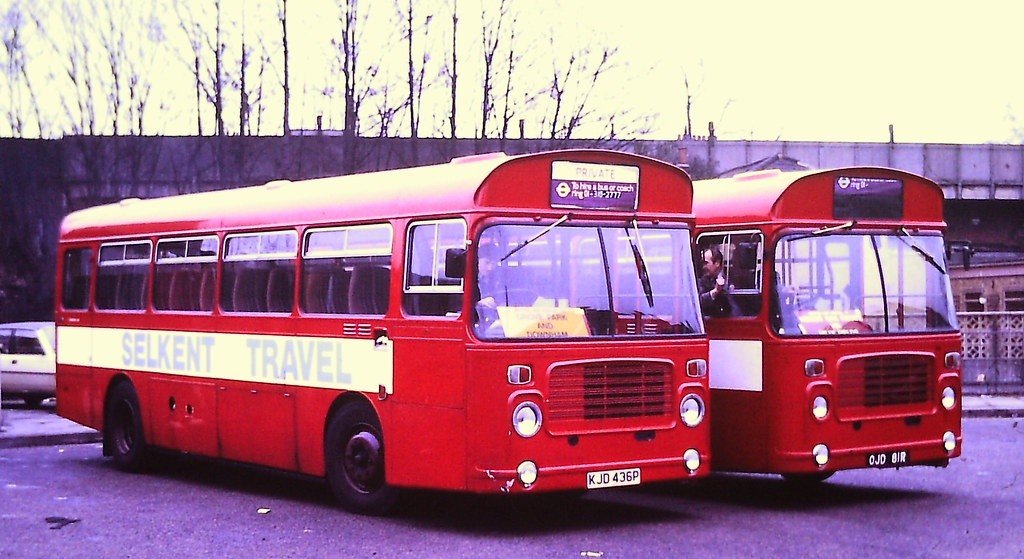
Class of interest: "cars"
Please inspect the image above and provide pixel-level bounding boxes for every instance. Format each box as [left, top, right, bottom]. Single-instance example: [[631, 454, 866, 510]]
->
[[0, 322, 55, 406]]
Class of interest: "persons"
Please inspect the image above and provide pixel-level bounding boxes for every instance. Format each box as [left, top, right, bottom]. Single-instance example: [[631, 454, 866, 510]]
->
[[697, 247, 761, 317]]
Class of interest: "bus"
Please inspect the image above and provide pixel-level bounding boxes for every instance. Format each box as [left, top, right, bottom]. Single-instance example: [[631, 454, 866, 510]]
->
[[434, 166, 975, 484], [54, 150, 712, 516]]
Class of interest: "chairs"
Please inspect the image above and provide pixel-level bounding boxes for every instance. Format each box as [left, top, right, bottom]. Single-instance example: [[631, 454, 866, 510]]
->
[[68, 262, 390, 314], [729, 246, 756, 289]]
